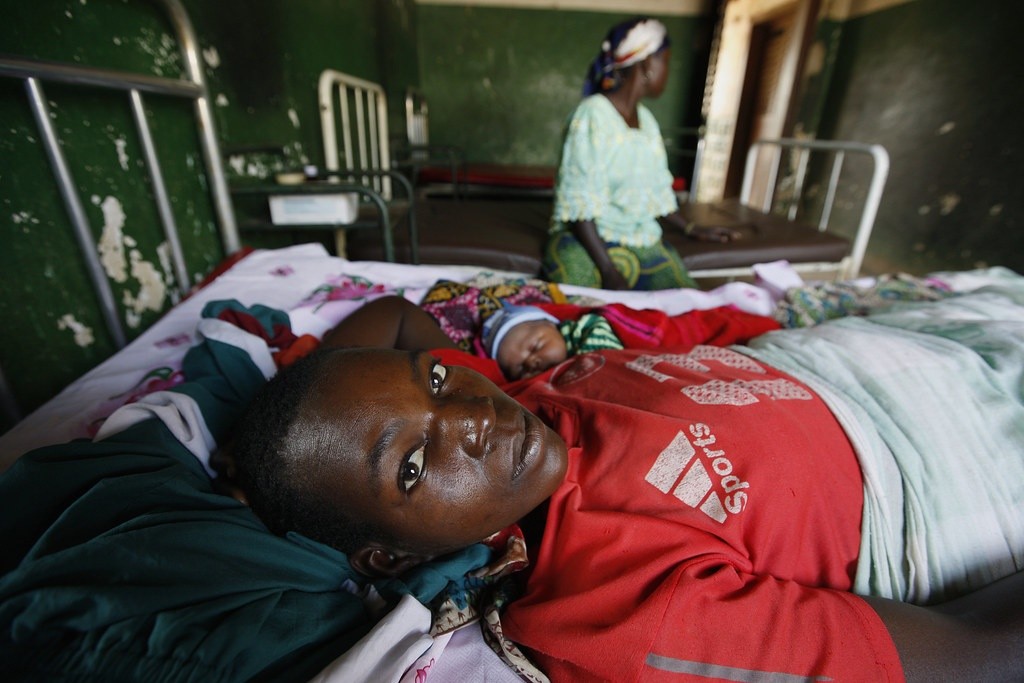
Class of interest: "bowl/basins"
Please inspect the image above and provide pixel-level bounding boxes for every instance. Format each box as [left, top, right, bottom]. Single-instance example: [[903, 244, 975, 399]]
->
[[274, 173, 306, 185]]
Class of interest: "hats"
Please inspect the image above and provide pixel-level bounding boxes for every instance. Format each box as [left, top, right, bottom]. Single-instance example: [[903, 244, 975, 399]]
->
[[482, 302, 560, 360]]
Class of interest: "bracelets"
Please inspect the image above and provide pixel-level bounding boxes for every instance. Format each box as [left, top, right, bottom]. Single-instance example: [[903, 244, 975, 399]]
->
[[686, 222, 695, 233]]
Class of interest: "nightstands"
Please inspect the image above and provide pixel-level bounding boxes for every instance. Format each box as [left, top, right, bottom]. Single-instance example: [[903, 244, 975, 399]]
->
[[228, 135, 464, 264]]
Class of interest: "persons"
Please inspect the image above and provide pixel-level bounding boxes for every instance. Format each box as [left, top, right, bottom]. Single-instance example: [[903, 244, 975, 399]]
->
[[540, 17, 741, 291], [209, 286, 1024, 683], [483, 304, 781, 380]]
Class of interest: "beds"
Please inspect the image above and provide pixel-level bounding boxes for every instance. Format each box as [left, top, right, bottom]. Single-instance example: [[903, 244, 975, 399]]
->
[[0, 0, 1024, 683], [404, 85, 689, 205], [318, 68, 890, 286]]
